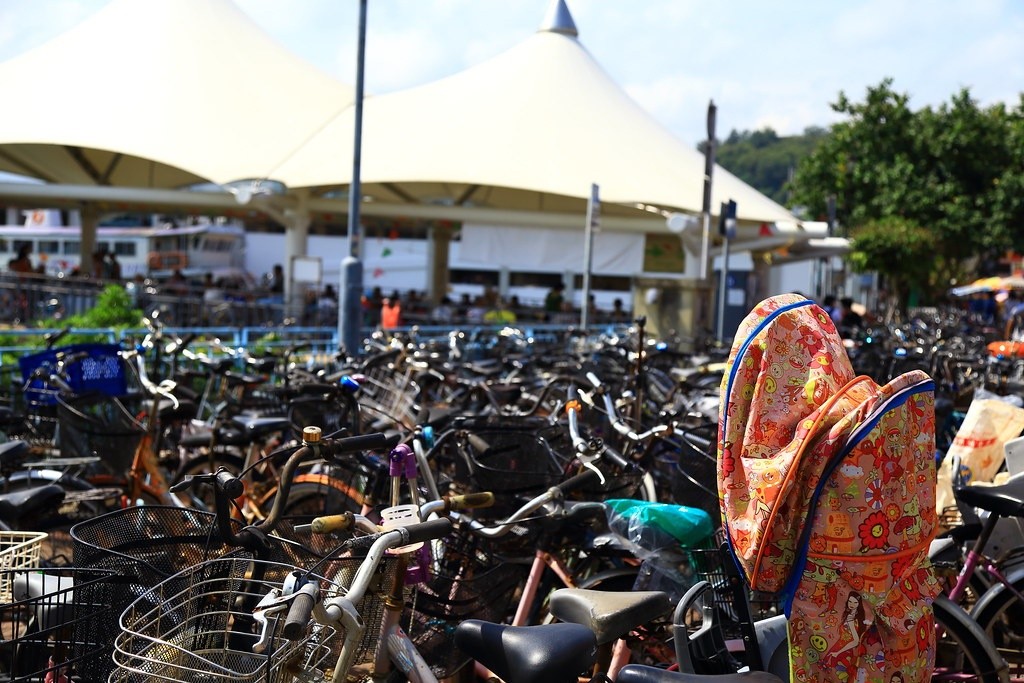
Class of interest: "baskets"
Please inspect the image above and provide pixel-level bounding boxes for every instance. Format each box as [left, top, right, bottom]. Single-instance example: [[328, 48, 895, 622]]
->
[[0, 344, 1024, 683]]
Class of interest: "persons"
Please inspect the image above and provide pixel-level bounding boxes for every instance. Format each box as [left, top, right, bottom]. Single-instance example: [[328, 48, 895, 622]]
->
[[818, 294, 860, 326], [7, 244, 629, 338], [968, 289, 1024, 341]]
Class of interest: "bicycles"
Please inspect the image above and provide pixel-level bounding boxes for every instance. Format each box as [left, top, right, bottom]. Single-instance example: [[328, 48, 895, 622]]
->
[[0, 301, 1023, 683]]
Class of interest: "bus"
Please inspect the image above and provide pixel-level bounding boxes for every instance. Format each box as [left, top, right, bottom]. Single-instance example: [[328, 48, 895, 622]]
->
[[0, 216, 250, 285]]
